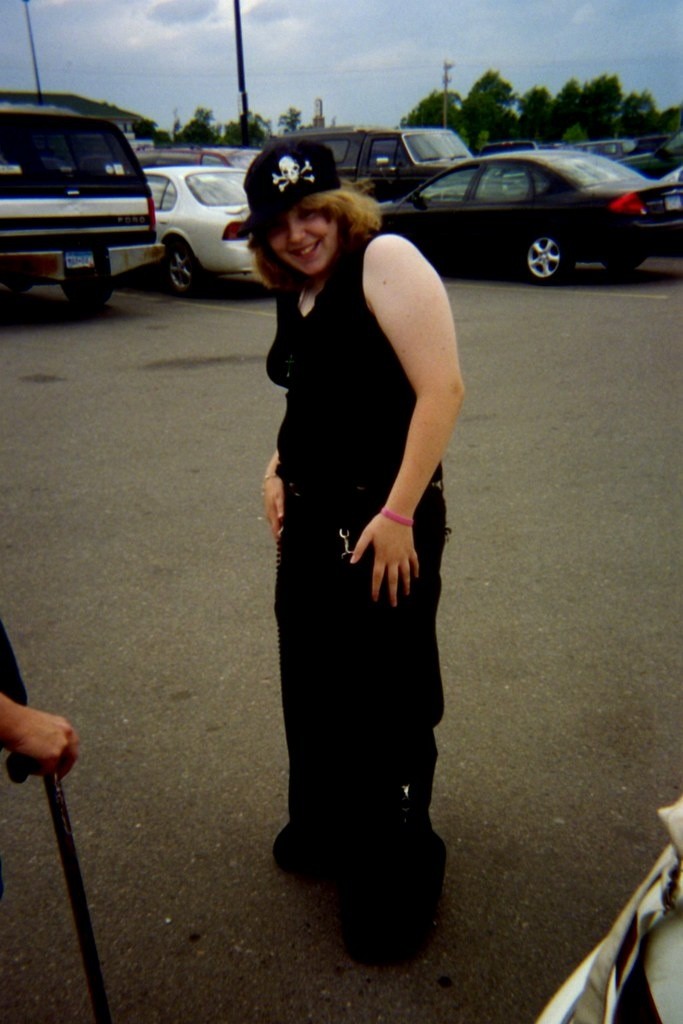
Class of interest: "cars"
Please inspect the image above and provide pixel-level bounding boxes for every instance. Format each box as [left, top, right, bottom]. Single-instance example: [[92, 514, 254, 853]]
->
[[372, 150, 683, 289], [479, 134, 683, 178], [140, 144, 261, 170], [140, 164, 273, 298]]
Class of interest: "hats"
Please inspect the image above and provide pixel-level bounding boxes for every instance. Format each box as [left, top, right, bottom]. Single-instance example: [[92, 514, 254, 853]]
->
[[218, 138, 341, 242]]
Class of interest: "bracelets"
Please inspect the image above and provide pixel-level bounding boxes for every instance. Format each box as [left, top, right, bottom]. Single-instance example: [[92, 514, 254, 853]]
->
[[380, 507, 416, 529], [260, 475, 274, 496]]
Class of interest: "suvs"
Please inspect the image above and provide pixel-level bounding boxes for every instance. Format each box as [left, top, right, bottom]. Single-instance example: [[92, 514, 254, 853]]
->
[[0, 107, 169, 314]]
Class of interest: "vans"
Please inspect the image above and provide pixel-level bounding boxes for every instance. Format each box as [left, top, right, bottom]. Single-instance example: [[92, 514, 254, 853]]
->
[[277, 129, 476, 204]]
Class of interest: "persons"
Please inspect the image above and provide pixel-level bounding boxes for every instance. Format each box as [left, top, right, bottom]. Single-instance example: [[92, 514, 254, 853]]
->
[[0, 616, 78, 904], [532, 793, 683, 1024], [223, 139, 466, 967]]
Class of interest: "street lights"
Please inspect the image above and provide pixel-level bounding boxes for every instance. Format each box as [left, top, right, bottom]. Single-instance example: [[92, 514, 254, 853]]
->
[[441, 58, 455, 130]]
